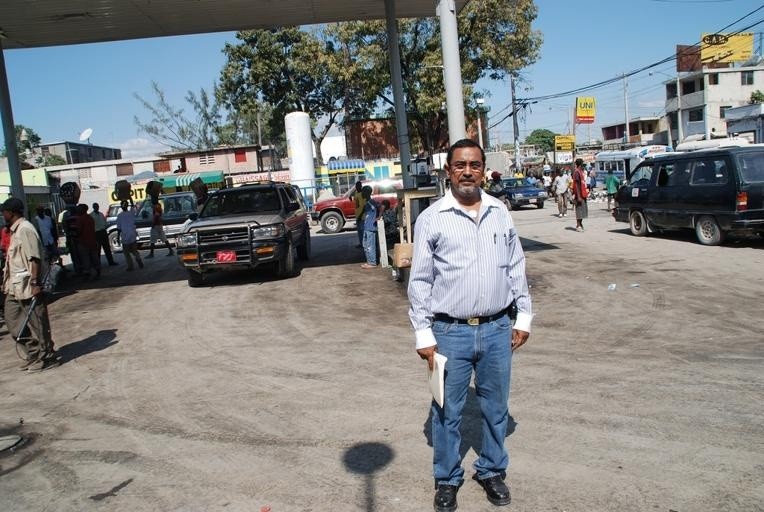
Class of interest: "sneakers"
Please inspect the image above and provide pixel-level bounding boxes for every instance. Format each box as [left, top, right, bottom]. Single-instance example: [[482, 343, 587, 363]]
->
[[18, 357, 60, 373]]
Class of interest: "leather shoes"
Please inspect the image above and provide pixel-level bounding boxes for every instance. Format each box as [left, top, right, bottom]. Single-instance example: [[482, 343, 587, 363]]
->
[[478, 473, 511, 505], [434, 484, 457, 512]]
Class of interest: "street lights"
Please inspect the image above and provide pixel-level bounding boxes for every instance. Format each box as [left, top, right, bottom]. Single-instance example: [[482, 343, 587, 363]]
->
[[472, 97, 484, 148], [648, 70, 683, 141], [547, 104, 571, 133]]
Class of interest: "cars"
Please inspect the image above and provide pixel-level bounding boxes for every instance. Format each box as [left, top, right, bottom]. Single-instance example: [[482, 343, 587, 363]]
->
[[485, 178, 548, 212], [104, 203, 133, 224]]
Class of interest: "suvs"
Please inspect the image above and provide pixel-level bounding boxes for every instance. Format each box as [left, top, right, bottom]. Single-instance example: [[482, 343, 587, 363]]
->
[[174, 179, 312, 289], [609, 143, 764, 247], [107, 188, 219, 254], [310, 176, 403, 235]]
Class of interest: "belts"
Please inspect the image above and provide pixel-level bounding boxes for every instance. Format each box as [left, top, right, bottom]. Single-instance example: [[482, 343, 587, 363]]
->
[[434, 308, 509, 324]]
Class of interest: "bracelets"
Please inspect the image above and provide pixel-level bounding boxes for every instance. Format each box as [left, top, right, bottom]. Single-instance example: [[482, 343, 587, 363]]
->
[[29, 277, 41, 287]]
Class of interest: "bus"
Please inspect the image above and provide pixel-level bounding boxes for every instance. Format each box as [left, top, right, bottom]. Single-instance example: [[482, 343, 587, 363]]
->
[[593, 144, 674, 186]]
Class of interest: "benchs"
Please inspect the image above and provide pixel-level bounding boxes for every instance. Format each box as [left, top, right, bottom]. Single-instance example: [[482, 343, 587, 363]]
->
[[670, 160, 728, 184]]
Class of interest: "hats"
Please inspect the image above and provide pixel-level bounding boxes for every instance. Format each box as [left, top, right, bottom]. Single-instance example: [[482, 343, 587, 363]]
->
[[0, 197, 25, 214]]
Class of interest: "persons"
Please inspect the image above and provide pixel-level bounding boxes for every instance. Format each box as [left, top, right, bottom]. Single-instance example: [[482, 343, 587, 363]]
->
[[349, 180, 366, 248], [381, 200, 402, 252], [356, 185, 385, 268], [143, 194, 174, 259], [193, 197, 206, 215], [571, 157, 592, 231], [30, 203, 97, 284], [89, 203, 119, 269], [481, 158, 626, 217], [405, 139, 534, 512], [0, 197, 62, 372], [0, 219, 11, 329], [114, 196, 145, 270]]
